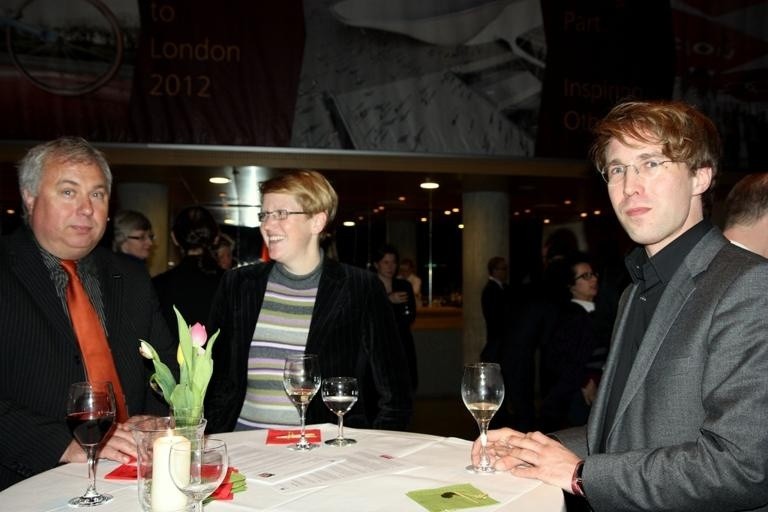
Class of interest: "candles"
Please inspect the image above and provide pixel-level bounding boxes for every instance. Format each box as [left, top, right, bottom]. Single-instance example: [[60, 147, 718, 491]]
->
[[151, 427, 191, 512]]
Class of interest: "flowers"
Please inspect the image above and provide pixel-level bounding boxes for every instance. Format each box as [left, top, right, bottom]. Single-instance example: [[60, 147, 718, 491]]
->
[[138, 305, 221, 461]]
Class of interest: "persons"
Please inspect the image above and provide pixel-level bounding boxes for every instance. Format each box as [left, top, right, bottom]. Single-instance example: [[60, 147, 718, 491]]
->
[[471, 99, 766, 512], [0, 135, 181, 491], [538, 226, 580, 431], [202, 167, 419, 437], [719, 166, 768, 261], [397, 257, 425, 311], [539, 253, 611, 434], [363, 239, 418, 416], [479, 254, 520, 429], [106, 206, 244, 345]]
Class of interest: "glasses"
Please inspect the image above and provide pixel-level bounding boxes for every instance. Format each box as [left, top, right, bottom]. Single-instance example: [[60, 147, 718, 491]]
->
[[127, 234, 153, 240], [575, 272, 599, 279], [259, 210, 306, 221], [601, 158, 675, 183]]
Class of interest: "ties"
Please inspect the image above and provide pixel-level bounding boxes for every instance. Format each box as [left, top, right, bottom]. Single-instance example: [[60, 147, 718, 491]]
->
[[60, 259, 130, 423]]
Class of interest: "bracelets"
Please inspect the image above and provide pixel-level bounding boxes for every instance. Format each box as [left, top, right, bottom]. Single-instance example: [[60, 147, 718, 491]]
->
[[571, 459, 588, 498]]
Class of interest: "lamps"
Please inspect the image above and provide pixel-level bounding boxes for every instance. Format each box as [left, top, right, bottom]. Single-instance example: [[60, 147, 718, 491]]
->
[[208, 168, 230, 184], [419, 178, 440, 190]]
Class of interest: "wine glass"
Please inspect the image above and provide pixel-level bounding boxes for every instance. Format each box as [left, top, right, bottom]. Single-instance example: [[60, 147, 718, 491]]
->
[[64, 381, 117, 507], [168, 439, 229, 512], [321, 378, 359, 445], [459, 362, 506, 476], [282, 355, 322, 450]]
